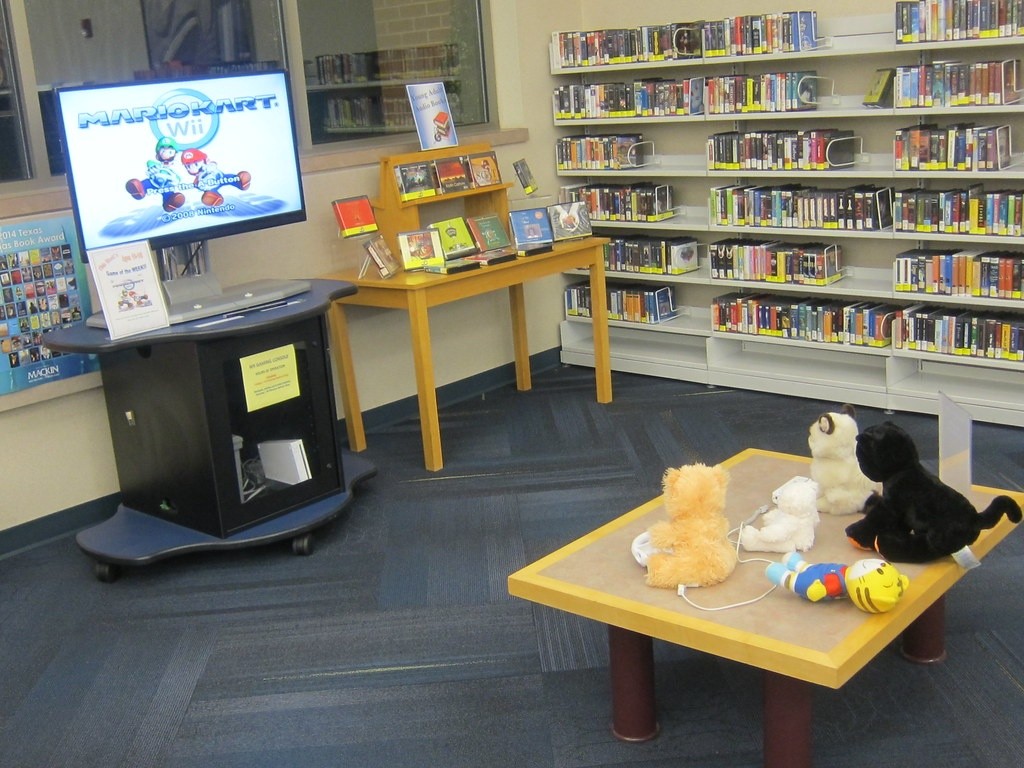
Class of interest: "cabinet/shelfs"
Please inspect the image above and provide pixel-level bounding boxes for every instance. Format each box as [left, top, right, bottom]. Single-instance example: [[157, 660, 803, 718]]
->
[[40, 280, 378, 581], [307, 76, 461, 133], [314, 143, 612, 471], [549, 11, 1024, 428]]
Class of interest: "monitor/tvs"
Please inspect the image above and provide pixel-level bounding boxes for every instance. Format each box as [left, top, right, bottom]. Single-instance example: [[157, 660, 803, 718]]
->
[[52, 68, 310, 328]]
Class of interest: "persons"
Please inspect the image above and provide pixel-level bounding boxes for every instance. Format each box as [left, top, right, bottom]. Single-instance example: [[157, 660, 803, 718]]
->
[[528, 228, 539, 238], [5, 289, 13, 301], [42, 347, 50, 360], [72, 307, 82, 322], [554, 201, 585, 233]]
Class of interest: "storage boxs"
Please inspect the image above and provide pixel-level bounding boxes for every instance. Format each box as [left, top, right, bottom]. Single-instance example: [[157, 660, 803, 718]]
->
[[231, 432, 244, 504], [257, 440, 313, 485]]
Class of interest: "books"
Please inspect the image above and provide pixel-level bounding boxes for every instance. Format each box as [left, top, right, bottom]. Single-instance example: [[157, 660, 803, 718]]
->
[[549, 0, 1024, 363], [394, 150, 594, 275], [315, 44, 461, 128], [363, 234, 402, 280], [331, 195, 378, 239]]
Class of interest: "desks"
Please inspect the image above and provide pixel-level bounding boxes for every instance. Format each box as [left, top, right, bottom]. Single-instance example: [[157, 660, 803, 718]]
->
[[508, 448, 1024, 768]]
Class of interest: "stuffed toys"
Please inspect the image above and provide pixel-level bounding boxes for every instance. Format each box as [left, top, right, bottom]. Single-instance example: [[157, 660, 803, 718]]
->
[[646, 464, 738, 590], [765, 551, 909, 613], [741, 482, 820, 553], [844, 422, 1022, 564], [808, 403, 875, 515]]
